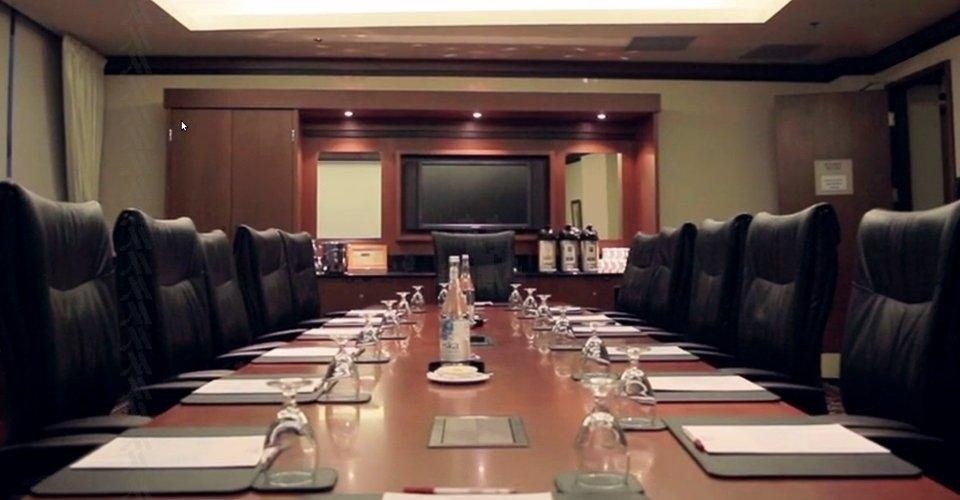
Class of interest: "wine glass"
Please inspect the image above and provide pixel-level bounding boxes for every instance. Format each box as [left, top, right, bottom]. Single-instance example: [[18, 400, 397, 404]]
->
[[259, 378, 320, 487], [532, 294, 554, 330], [548, 305, 582, 350], [356, 309, 383, 362], [322, 330, 364, 401], [378, 285, 425, 338], [576, 319, 617, 387], [508, 283, 537, 319], [573, 370, 633, 491], [610, 344, 658, 429]]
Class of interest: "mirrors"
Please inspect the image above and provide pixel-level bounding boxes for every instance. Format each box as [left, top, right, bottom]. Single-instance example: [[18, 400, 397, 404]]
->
[[564, 152, 625, 241]]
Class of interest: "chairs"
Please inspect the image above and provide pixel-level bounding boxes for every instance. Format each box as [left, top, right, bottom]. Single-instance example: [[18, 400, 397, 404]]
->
[[431, 230, 515, 303]]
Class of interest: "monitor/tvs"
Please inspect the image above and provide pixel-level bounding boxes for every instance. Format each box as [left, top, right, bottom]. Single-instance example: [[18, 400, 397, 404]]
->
[[417, 161, 532, 230]]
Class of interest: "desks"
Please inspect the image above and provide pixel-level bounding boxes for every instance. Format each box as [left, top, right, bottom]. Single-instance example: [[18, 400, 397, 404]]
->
[[22, 303, 958, 498]]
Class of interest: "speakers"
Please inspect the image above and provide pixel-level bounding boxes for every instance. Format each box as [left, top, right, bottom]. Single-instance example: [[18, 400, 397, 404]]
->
[[405, 161, 418, 230], [532, 161, 546, 230]]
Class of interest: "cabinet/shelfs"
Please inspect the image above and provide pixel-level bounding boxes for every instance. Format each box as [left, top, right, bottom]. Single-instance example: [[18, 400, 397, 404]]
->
[[165, 107, 301, 253]]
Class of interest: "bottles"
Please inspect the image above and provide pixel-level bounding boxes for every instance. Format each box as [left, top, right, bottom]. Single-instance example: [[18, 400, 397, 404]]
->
[[537, 225, 559, 274], [577, 224, 600, 273], [557, 224, 582, 273], [439, 253, 475, 362]]
